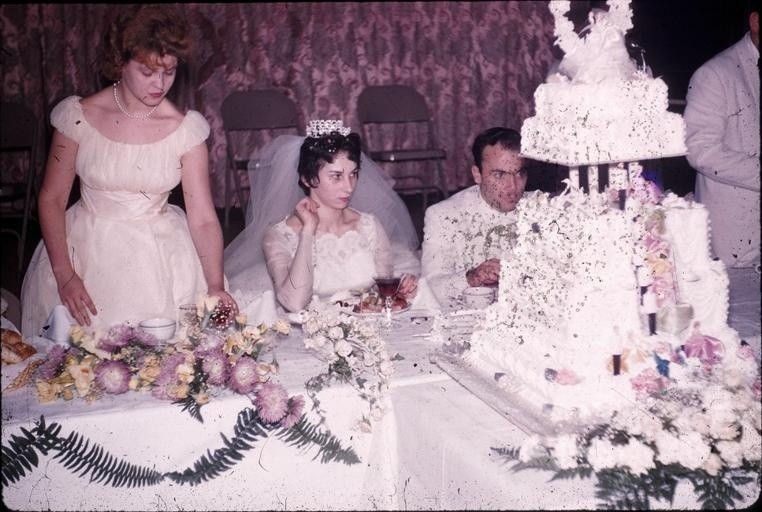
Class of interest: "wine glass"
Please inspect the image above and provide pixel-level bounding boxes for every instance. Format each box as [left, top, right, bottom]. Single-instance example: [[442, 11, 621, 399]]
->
[[207, 300, 232, 340], [372, 272, 402, 330]]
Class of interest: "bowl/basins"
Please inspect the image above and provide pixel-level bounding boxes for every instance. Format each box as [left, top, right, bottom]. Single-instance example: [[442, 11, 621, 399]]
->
[[462, 286, 493, 311], [139, 316, 174, 339]]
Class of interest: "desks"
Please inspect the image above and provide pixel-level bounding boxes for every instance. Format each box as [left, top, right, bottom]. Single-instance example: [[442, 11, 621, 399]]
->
[[0, 269, 762, 431]]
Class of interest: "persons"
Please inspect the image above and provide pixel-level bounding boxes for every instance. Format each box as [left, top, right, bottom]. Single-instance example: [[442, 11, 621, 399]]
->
[[19, 5, 238, 346], [221, 119, 422, 323], [681, 8, 760, 269], [414, 127, 545, 313]]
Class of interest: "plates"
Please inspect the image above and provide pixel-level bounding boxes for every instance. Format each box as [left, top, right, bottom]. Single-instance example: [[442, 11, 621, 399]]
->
[[330, 289, 411, 317]]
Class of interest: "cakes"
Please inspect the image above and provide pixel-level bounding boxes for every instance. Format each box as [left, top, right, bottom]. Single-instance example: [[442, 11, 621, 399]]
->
[[466, 0, 729, 422]]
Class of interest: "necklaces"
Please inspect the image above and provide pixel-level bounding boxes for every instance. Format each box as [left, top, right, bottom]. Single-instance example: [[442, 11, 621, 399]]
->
[[112, 80, 159, 119]]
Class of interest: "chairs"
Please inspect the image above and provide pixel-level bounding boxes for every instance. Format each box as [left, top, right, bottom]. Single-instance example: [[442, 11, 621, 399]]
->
[[0, 104, 41, 275], [356, 83, 451, 217], [220, 89, 311, 229]]
